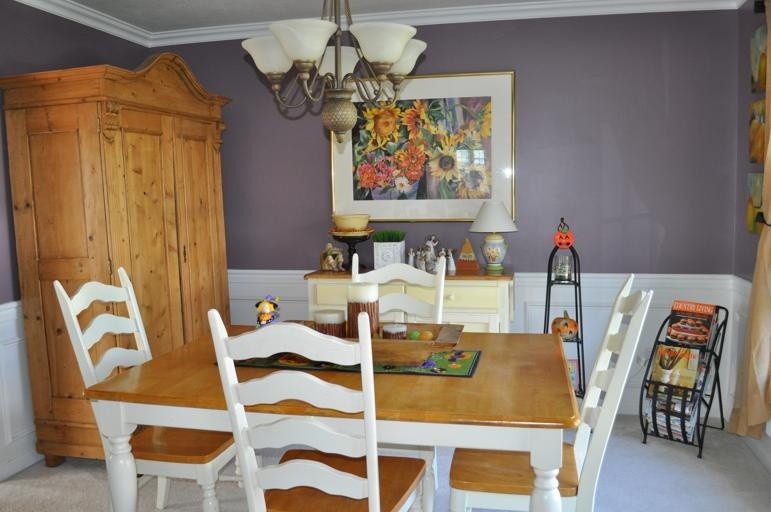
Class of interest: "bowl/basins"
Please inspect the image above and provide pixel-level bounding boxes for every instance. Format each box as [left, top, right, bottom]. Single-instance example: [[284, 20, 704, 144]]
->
[[333, 214, 370, 231]]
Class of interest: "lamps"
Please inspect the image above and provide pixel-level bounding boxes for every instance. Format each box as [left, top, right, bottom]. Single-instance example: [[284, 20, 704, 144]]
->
[[468, 200, 519, 271], [241, 0, 428, 143]]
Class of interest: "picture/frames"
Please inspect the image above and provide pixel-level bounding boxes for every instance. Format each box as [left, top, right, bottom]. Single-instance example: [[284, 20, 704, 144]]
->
[[330, 70, 516, 223]]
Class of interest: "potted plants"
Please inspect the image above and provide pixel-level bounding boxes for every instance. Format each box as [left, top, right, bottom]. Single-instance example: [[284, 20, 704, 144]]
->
[[373, 229, 406, 270]]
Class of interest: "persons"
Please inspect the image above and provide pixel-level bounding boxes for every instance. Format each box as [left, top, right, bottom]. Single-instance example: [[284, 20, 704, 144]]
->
[[252, 295, 280, 329], [320, 234, 457, 277]]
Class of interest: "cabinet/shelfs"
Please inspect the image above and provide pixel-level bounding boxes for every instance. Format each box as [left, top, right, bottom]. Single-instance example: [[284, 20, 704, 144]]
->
[[3, 50, 231, 469]]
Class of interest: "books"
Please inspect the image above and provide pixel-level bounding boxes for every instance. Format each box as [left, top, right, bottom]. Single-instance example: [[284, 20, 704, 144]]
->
[[645, 298, 720, 442]]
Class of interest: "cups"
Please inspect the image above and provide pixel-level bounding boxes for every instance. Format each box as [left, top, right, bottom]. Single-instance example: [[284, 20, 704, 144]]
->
[[553, 253, 573, 282]]
